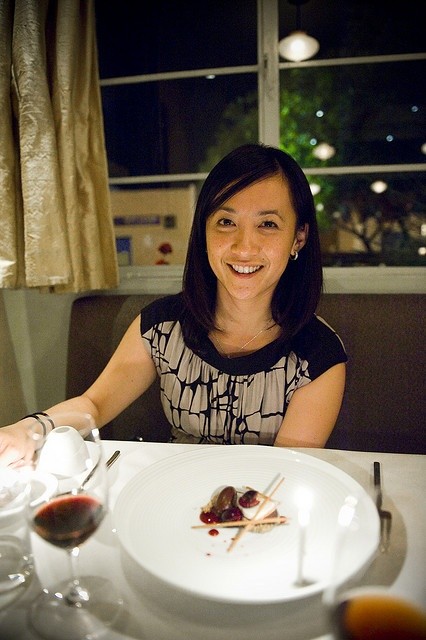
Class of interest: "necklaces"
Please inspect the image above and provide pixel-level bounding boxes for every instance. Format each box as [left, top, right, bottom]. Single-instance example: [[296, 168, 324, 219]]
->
[[211, 318, 272, 359]]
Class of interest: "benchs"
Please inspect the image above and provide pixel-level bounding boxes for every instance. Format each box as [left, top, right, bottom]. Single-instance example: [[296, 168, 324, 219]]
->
[[66, 295, 426, 454]]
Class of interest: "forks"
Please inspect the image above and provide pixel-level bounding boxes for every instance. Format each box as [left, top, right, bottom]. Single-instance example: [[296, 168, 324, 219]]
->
[[373, 461, 393, 555]]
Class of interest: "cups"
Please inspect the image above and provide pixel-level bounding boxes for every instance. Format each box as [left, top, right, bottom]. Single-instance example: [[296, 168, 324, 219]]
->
[[37, 425, 91, 480]]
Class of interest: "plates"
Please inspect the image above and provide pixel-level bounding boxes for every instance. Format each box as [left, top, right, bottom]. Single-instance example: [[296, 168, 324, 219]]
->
[[113, 444, 382, 607]]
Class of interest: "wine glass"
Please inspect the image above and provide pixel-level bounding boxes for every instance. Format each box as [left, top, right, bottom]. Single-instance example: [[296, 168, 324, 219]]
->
[[26, 413, 123, 640]]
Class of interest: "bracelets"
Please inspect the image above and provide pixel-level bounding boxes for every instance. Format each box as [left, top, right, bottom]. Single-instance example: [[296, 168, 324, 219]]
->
[[21, 415, 46, 438], [0, 143, 348, 468], [32, 412, 55, 430]]
